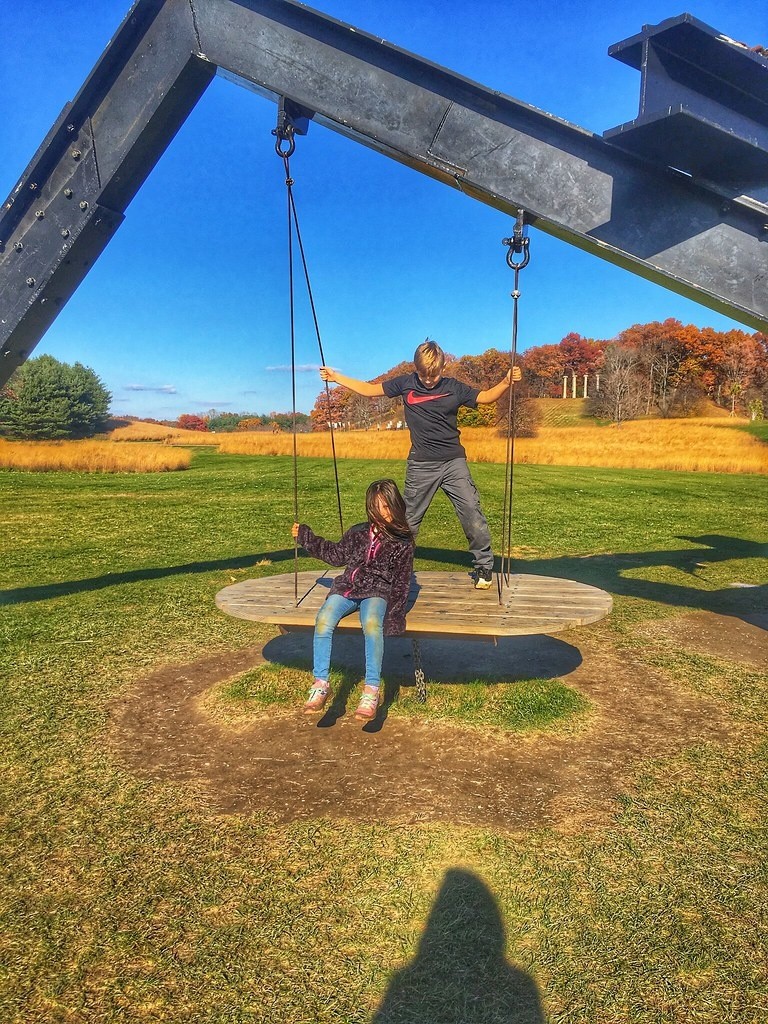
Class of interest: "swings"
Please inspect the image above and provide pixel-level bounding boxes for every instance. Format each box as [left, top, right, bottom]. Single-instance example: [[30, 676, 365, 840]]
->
[[212, 151, 614, 637]]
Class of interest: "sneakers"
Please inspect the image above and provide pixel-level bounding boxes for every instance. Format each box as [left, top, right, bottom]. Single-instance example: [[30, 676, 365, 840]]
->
[[303, 681, 334, 714], [353, 685, 383, 722], [474, 565, 492, 590]]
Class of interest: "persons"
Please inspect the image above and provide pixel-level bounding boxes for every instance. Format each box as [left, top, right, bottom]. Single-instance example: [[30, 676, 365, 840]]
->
[[291, 479, 415, 722], [319, 340, 522, 591]]
